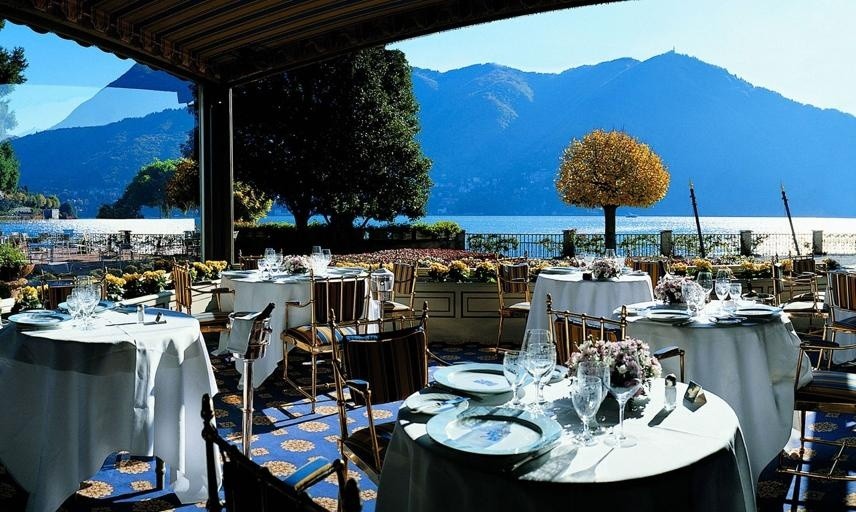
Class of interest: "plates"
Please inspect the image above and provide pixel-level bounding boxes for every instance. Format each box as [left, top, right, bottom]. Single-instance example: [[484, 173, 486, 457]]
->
[[433, 363, 534, 394], [25, 309, 55, 315], [540, 267, 574, 274], [56, 300, 117, 314], [709, 317, 747, 324], [408, 393, 470, 415], [731, 305, 782, 317], [337, 267, 365, 273], [647, 308, 690, 321], [221, 271, 257, 277], [7, 311, 70, 328], [427, 406, 563, 455]]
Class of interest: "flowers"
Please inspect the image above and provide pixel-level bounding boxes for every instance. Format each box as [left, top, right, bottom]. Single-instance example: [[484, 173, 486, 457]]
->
[[561, 332, 667, 397]]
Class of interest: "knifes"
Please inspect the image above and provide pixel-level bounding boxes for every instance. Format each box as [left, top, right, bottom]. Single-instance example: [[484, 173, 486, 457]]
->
[[410, 397, 471, 414], [503, 440, 562, 474]]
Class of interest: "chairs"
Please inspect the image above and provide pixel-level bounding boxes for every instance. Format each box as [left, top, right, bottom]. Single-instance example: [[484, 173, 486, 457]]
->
[[325, 301, 456, 490], [770, 319, 855, 482], [33, 248, 425, 415], [488, 247, 856, 355], [199, 391, 366, 510], [543, 290, 687, 385]]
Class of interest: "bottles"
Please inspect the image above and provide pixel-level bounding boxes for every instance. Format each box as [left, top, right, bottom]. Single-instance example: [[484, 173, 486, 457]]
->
[[665, 374, 677, 412], [136, 303, 145, 326]]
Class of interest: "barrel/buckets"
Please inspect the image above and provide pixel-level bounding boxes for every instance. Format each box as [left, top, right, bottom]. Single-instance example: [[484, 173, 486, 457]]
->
[[371, 262, 394, 299], [742, 291, 777, 308], [228, 311, 272, 361]]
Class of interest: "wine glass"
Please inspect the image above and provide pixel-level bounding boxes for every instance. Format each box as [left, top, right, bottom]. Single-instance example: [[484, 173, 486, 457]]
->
[[603, 361, 643, 448], [500, 328, 611, 446], [257, 245, 332, 280], [576, 247, 617, 272], [65, 275, 102, 332], [683, 269, 742, 317]]
